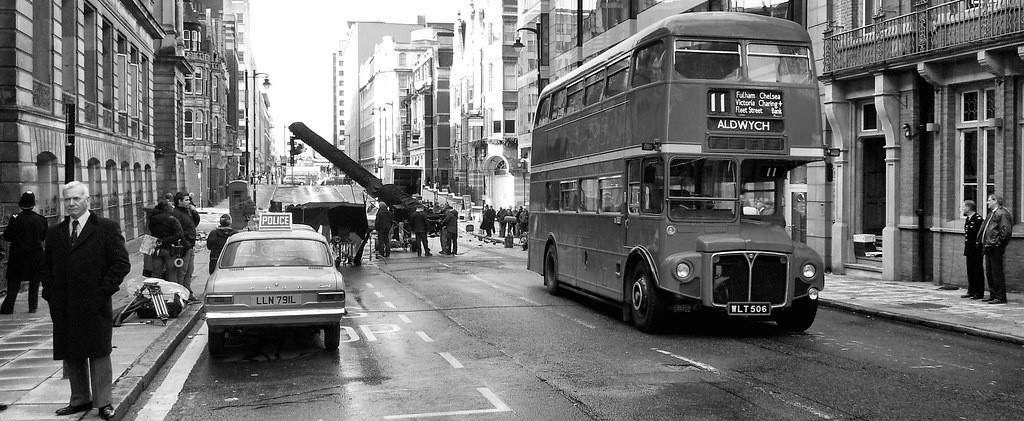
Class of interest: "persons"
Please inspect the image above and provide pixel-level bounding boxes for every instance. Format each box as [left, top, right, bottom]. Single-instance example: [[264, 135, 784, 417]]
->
[[480, 205, 528, 252], [43, 181, 131, 418], [270, 200, 303, 227], [375, 199, 458, 256], [977, 194, 1014, 304], [961, 200, 984, 300], [0, 191, 49, 315], [149, 191, 241, 307]]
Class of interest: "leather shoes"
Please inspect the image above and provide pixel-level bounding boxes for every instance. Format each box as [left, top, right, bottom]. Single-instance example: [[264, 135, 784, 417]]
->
[[56, 400, 93, 415], [99, 402, 115, 418]]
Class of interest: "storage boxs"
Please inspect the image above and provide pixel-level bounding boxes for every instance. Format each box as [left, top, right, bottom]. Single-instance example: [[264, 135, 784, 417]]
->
[[854, 234, 877, 256]]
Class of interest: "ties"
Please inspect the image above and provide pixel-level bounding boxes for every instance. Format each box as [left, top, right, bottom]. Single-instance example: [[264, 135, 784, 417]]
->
[[70, 220, 79, 245]]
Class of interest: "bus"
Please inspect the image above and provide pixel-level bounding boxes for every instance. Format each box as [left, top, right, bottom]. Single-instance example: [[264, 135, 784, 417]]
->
[[527, 15, 835, 334]]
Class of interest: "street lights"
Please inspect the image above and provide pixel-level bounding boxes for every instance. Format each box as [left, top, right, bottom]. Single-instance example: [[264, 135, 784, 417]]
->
[[370, 106, 383, 181], [383, 101, 395, 164], [251, 68, 271, 206], [509, 21, 542, 100]]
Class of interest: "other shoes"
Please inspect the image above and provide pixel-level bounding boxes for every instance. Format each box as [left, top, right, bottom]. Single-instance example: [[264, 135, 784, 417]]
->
[[189, 297, 201, 304], [425, 253, 433, 256], [29, 308, 36, 313], [452, 251, 456, 255], [439, 251, 446, 254]]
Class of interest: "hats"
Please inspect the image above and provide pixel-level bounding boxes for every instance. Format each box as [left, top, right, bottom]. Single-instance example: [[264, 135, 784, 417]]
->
[[18, 191, 35, 209]]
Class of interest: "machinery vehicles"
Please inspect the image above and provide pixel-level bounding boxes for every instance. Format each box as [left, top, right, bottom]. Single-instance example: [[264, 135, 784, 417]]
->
[[288, 121, 447, 251]]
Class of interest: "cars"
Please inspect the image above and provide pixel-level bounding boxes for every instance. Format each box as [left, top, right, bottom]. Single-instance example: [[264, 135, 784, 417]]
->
[[203, 211, 348, 355], [282, 176, 307, 186]]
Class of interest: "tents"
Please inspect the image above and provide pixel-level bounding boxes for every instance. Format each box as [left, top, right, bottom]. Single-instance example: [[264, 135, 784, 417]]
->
[[291, 202, 369, 266]]
